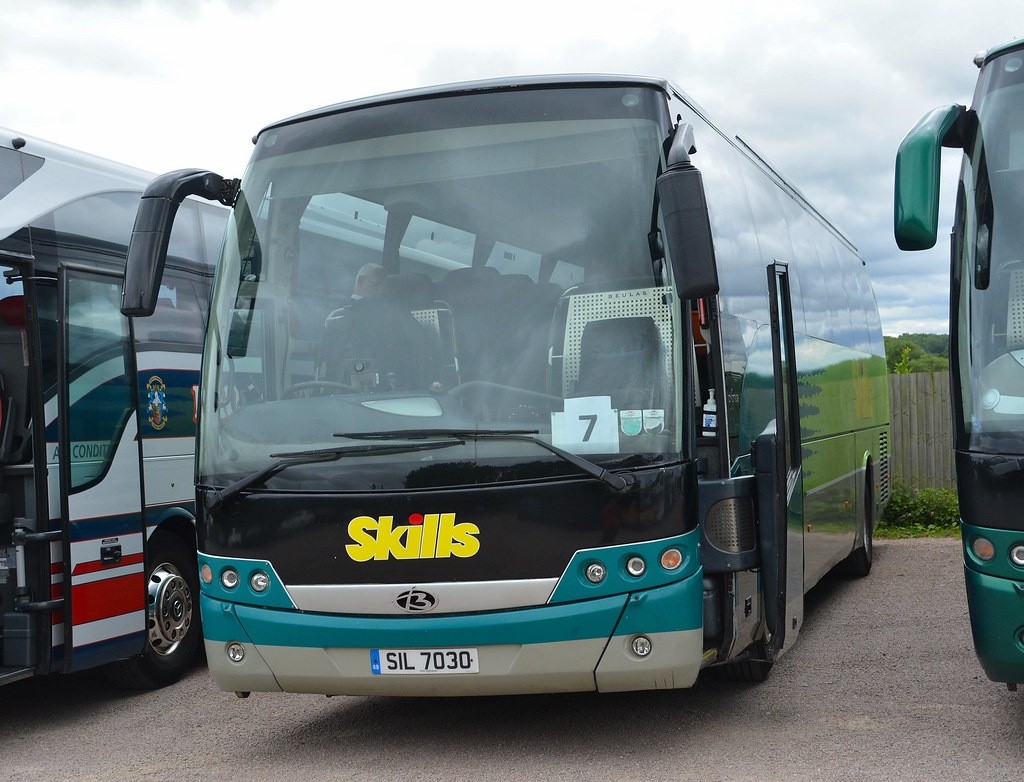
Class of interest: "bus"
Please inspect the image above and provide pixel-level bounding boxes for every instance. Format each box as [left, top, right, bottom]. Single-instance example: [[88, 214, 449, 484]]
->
[[894, 39, 1024, 691], [0, 126, 472, 685], [120, 73, 894, 698]]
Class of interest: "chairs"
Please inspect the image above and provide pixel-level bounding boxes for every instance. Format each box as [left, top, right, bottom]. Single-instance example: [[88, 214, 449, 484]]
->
[[0, 324, 32, 466], [386, 267, 563, 311], [576, 316, 661, 414]]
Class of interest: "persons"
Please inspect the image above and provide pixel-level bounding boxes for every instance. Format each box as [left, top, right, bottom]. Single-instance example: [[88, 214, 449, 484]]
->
[[340, 262, 390, 305]]
